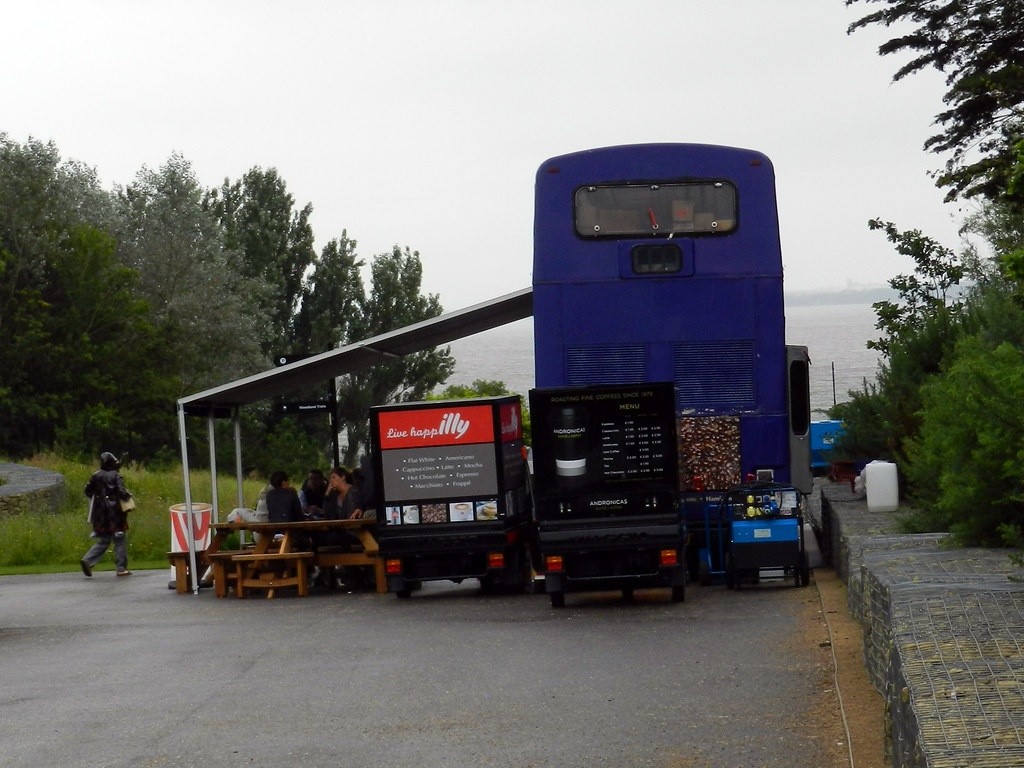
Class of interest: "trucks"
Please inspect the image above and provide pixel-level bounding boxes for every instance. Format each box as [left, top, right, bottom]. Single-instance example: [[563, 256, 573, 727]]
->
[[369, 394, 533, 596], [531, 378, 691, 608]]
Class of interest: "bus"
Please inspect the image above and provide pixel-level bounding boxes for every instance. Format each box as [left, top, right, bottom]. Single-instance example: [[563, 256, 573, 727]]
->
[[531, 141, 810, 580]]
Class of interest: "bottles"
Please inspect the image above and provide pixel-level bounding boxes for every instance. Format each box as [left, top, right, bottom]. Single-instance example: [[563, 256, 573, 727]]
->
[[390, 507, 399, 524], [551, 407, 586, 469]]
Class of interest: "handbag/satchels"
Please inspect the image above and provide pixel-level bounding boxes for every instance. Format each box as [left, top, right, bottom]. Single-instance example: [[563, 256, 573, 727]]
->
[[97, 497, 121, 519]]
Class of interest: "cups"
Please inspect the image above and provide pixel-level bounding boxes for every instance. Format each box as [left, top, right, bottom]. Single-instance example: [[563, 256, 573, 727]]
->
[[455, 503, 473, 520], [408, 510, 418, 521]]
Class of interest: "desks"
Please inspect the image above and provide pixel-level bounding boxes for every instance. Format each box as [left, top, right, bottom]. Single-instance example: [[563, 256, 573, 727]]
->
[[200, 518, 387, 593]]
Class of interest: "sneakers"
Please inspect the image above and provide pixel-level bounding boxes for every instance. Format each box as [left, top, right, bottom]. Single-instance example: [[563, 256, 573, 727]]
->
[[80, 559, 92, 577], [117, 570, 131, 576]]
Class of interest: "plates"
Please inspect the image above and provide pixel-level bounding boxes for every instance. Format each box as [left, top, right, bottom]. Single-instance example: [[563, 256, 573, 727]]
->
[[404, 514, 419, 523], [476, 504, 497, 519]]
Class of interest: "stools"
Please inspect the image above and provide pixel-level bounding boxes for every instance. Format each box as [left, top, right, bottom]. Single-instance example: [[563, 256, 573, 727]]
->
[[208, 551, 250, 597], [366, 549, 387, 592], [233, 552, 314, 598], [166, 550, 209, 594]]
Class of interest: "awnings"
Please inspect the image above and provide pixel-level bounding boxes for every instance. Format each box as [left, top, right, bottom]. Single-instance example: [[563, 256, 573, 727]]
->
[[176, 287, 534, 415]]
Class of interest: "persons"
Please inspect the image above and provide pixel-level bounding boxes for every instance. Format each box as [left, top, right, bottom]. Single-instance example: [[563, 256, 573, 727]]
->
[[81, 452, 134, 577], [254, 465, 377, 596]]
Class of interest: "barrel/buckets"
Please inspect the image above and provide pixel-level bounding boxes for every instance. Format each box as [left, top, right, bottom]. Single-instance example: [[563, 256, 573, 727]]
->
[[865, 462, 899, 512]]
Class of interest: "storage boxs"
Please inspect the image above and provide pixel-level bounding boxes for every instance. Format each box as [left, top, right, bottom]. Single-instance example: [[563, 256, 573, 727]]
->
[[672, 199, 695, 223]]
